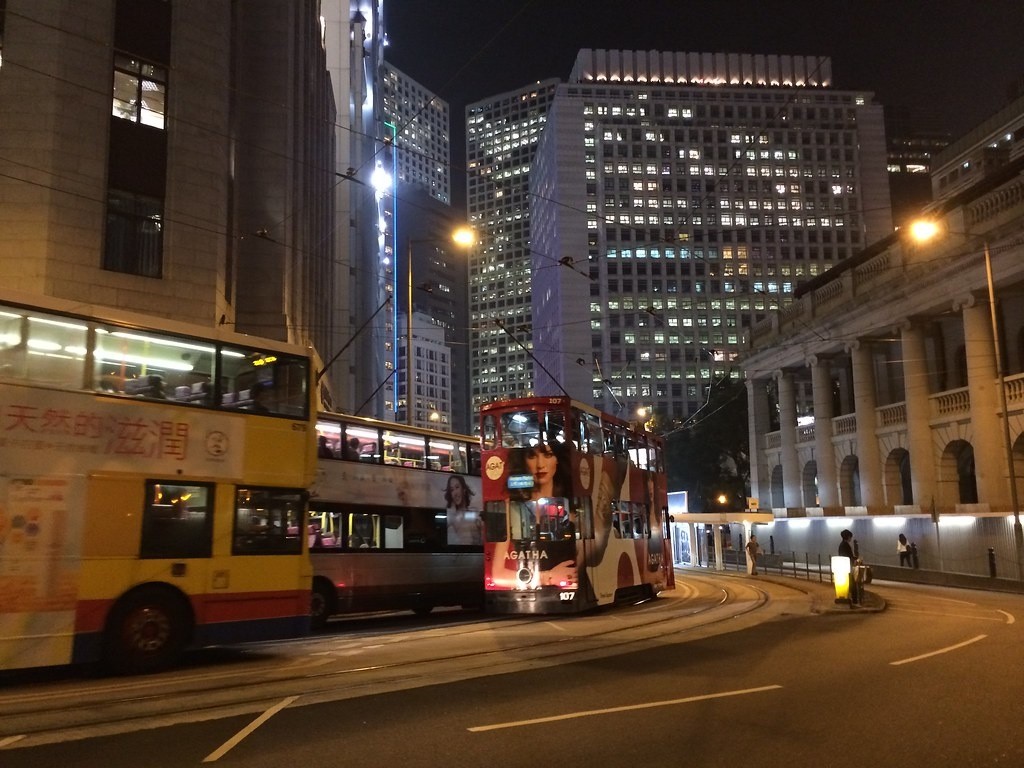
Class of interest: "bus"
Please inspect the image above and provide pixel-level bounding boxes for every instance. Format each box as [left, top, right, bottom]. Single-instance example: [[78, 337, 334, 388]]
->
[[0, 293, 318, 682], [311, 410, 481, 627], [481, 398, 675, 614]]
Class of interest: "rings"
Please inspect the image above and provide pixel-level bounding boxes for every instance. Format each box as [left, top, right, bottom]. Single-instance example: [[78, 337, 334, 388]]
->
[[567, 581, 572, 585], [567, 575, 572, 579]]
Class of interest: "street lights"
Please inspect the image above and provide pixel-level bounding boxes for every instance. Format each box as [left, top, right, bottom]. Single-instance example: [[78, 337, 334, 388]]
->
[[900, 216, 1024, 582]]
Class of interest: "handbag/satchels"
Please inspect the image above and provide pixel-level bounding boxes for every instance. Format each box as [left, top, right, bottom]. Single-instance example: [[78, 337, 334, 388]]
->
[[907, 545, 912, 555]]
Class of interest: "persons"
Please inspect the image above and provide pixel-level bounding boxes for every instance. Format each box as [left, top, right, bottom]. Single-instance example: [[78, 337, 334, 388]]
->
[[491, 443, 578, 589], [318, 435, 334, 458], [248, 384, 269, 414], [746, 535, 763, 575], [897, 534, 912, 567], [642, 472, 671, 588], [838, 530, 861, 609], [347, 438, 360, 461], [442, 475, 481, 545]]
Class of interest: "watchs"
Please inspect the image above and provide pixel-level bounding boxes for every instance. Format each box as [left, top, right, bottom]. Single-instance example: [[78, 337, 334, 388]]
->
[[517, 567, 532, 589]]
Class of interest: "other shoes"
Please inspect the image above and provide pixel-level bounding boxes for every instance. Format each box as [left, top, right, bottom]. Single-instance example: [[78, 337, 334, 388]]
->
[[751, 572, 758, 575]]
